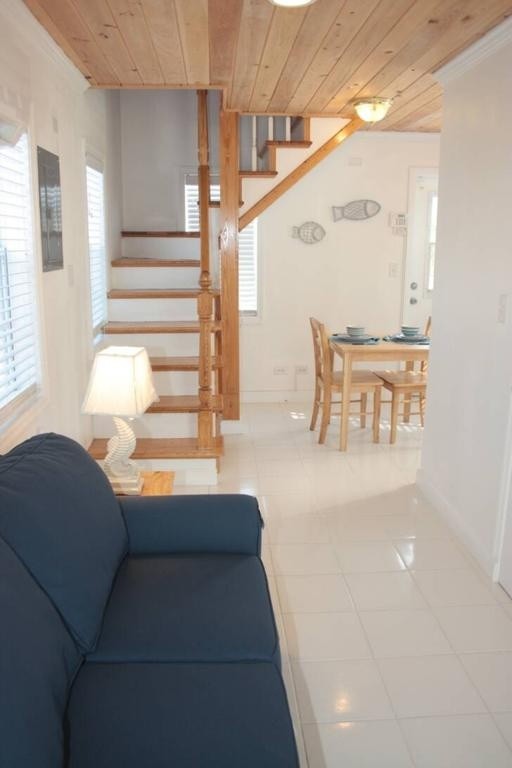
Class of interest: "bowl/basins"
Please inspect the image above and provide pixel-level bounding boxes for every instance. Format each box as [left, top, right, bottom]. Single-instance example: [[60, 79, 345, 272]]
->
[[346, 325, 365, 337], [401, 324, 421, 336]]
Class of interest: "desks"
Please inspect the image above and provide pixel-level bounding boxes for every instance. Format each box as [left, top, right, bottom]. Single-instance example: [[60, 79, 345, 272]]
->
[[328, 332, 432, 452], [115, 470, 176, 496]]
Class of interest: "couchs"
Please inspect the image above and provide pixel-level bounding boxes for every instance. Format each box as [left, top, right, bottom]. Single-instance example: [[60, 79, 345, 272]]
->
[[0, 432, 302, 768]]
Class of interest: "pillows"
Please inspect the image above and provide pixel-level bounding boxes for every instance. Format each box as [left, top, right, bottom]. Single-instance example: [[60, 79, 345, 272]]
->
[[0, 433, 130, 657], [0, 534, 87, 768]]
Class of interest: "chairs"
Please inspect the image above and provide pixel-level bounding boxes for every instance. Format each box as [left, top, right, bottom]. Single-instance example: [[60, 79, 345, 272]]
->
[[373, 315, 430, 444], [308, 316, 383, 445]]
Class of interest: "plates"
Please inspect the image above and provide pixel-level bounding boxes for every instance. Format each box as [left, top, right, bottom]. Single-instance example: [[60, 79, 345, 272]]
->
[[338, 334, 373, 341], [391, 333, 426, 341]]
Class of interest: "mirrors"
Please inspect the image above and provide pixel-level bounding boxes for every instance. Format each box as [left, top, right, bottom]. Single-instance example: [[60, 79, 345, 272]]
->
[[36, 142, 63, 272]]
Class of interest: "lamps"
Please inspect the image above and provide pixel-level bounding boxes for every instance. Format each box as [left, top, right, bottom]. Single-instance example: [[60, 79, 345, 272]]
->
[[353, 97, 394, 125], [264, 0, 316, 9], [81, 345, 160, 497]]
